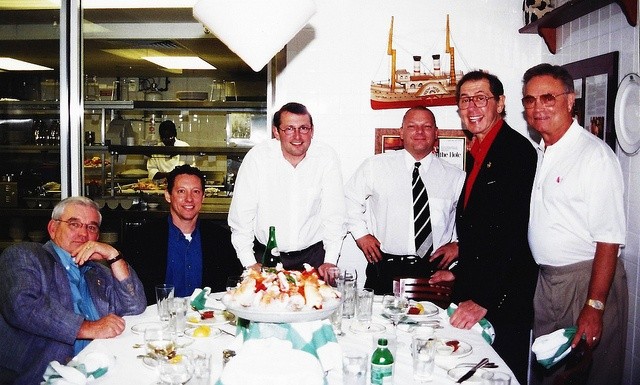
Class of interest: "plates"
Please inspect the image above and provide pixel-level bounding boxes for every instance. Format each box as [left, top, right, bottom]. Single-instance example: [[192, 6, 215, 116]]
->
[[447, 366, 494, 383], [157, 363, 192, 385], [183, 308, 237, 326], [433, 337, 474, 359], [613, 72, 640, 156], [221, 345, 325, 385], [130, 321, 170, 335], [220, 286, 345, 325], [349, 322, 387, 336], [183, 325, 222, 339], [383, 301, 439, 319], [101, 232, 119, 244]]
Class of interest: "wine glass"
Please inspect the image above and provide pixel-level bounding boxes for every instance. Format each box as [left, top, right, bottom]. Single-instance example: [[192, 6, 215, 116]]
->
[[166, 296, 188, 338], [337, 268, 358, 291], [380, 293, 411, 346], [326, 266, 337, 290]]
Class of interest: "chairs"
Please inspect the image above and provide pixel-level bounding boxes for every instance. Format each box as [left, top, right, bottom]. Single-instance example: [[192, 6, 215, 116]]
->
[[398, 278, 450, 311]]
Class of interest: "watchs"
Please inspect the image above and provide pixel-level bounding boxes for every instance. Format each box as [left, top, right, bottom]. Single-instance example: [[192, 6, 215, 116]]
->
[[585, 299, 607, 312]]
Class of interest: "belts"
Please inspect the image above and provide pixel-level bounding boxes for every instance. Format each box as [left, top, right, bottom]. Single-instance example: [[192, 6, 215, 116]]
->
[[254, 236, 326, 258], [374, 251, 440, 266]]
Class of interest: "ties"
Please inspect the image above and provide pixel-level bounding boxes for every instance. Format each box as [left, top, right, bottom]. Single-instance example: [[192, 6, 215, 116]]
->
[[412, 162, 433, 261]]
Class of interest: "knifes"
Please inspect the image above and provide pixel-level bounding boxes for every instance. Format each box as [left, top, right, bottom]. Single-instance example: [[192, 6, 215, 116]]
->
[[456, 358, 489, 383]]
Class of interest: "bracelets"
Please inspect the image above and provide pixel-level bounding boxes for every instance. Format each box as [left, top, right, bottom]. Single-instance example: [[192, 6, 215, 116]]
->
[[107, 254, 123, 264]]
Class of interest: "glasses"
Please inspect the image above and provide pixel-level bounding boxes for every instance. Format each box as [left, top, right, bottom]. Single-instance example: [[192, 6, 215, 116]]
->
[[457, 95, 500, 108], [276, 125, 312, 135], [54, 218, 100, 234], [522, 92, 570, 107]]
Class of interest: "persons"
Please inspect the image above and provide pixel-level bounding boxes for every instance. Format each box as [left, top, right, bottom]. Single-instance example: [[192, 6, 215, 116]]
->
[[147, 120, 196, 182], [429, 70, 537, 385], [128, 164, 246, 306], [521, 62, 629, 384], [347, 107, 466, 296], [0, 196, 147, 385], [227, 102, 347, 283]]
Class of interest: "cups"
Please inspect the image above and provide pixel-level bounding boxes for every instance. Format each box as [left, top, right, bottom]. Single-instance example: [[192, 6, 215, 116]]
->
[[341, 285, 356, 319], [481, 370, 511, 385], [185, 351, 212, 385], [410, 333, 438, 384], [342, 351, 369, 385], [154, 283, 175, 322], [327, 300, 346, 337], [355, 288, 374, 320]]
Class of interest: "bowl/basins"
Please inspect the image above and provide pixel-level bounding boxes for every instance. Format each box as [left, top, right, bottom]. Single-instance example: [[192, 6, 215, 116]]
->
[[8, 217, 27, 241], [27, 229, 49, 242], [148, 203, 160, 207], [175, 91, 208, 102]]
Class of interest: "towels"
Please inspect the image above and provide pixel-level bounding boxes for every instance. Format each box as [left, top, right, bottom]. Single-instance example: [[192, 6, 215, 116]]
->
[[41, 360, 109, 385], [187, 287, 213, 311], [445, 300, 496, 346], [532, 325, 589, 369]]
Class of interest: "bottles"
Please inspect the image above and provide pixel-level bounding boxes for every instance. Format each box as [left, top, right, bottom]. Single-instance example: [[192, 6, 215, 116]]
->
[[370, 338, 395, 385], [261, 226, 284, 269]]
